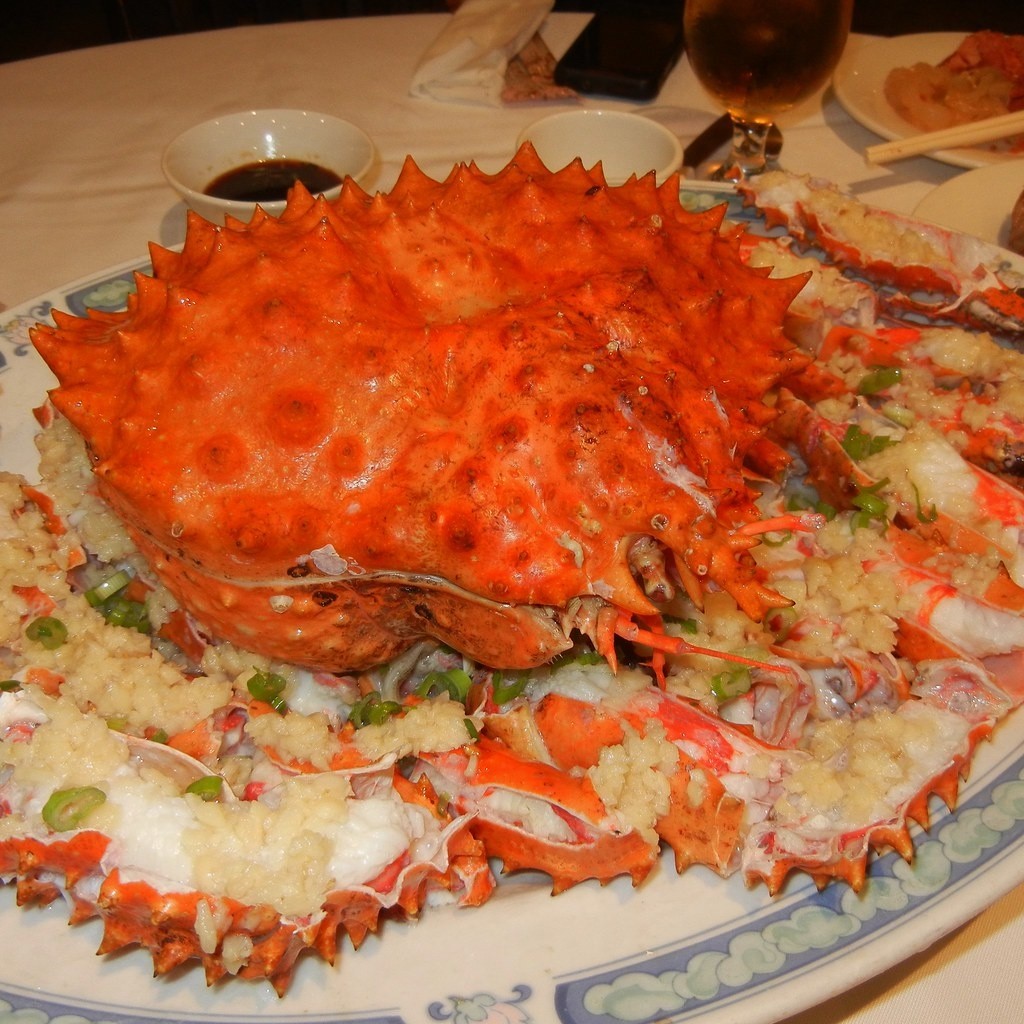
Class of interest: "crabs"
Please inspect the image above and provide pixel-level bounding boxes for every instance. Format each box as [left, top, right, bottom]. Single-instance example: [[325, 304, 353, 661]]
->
[[28, 144, 858, 683]]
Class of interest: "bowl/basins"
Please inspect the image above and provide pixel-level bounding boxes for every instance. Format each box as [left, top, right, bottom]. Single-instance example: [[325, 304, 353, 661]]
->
[[162, 107, 376, 225], [518, 109, 684, 188]]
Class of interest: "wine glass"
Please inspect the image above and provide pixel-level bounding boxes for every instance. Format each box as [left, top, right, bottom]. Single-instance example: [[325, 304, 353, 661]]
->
[[679, 0, 851, 183]]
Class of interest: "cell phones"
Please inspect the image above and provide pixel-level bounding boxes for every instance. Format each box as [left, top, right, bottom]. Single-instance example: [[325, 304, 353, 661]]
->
[[551, 0, 689, 102]]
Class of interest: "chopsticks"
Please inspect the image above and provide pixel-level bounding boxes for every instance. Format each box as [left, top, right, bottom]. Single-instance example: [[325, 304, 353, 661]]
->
[[862, 111, 1024, 165]]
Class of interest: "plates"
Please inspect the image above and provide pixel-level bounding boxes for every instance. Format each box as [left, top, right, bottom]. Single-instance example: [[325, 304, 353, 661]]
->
[[0, 182, 1023, 1023], [830, 30, 1024, 169], [907, 164, 1024, 249]]
